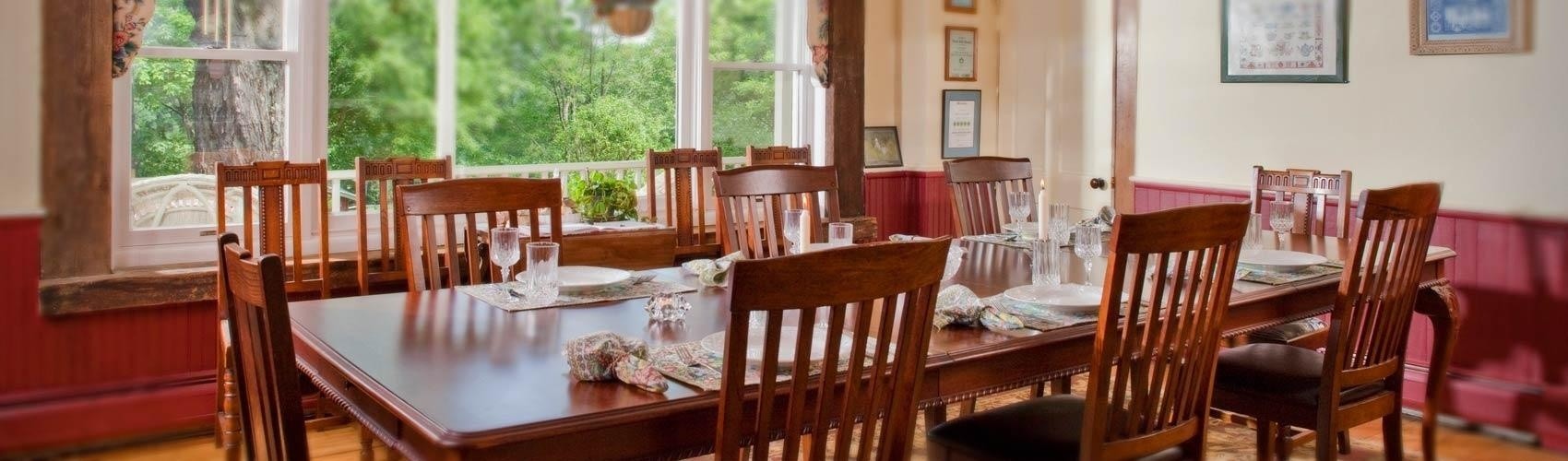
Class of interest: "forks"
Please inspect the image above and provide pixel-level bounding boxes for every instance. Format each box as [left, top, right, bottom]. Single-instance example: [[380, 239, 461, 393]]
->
[[631, 275, 655, 287], [675, 346, 723, 383]]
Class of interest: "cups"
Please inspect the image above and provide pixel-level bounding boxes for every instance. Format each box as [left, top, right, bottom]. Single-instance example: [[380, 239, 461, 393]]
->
[[1048, 203, 1069, 246], [1240, 210, 1263, 252], [828, 223, 853, 247], [526, 241, 561, 304], [1032, 239, 1061, 283]]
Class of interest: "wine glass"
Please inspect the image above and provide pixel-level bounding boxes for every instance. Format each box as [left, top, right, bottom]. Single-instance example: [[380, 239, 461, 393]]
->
[[1073, 224, 1103, 288], [783, 209, 809, 254], [1268, 200, 1295, 249], [490, 225, 521, 307], [1006, 190, 1031, 245]]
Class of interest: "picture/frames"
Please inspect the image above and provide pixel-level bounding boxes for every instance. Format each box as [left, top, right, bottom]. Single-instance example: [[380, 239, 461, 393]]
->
[[1218, 1, 1352, 87], [944, 0, 977, 15], [939, 88, 984, 160], [1406, 0, 1538, 60], [945, 25, 980, 84], [862, 122, 904, 170]]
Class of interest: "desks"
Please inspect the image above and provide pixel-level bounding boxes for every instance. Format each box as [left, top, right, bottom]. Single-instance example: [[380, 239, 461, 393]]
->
[[288, 220, 1458, 460]]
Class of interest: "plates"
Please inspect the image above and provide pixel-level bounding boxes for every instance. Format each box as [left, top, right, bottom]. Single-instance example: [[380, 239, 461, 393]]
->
[[1001, 219, 1077, 236], [1229, 248, 1327, 271], [699, 325, 851, 366], [514, 264, 631, 296], [1003, 284, 1129, 310], [804, 242, 830, 253]]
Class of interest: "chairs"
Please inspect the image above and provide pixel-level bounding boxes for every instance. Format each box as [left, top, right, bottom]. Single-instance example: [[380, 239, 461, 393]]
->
[[714, 235, 953, 458], [396, 178, 564, 291], [708, 163, 844, 262], [926, 197, 1254, 461], [646, 145, 725, 258], [1250, 162, 1355, 459], [746, 143, 825, 252], [215, 230, 311, 460], [1177, 178, 1446, 460], [213, 154, 331, 302], [944, 154, 1048, 416], [351, 154, 455, 292]]
